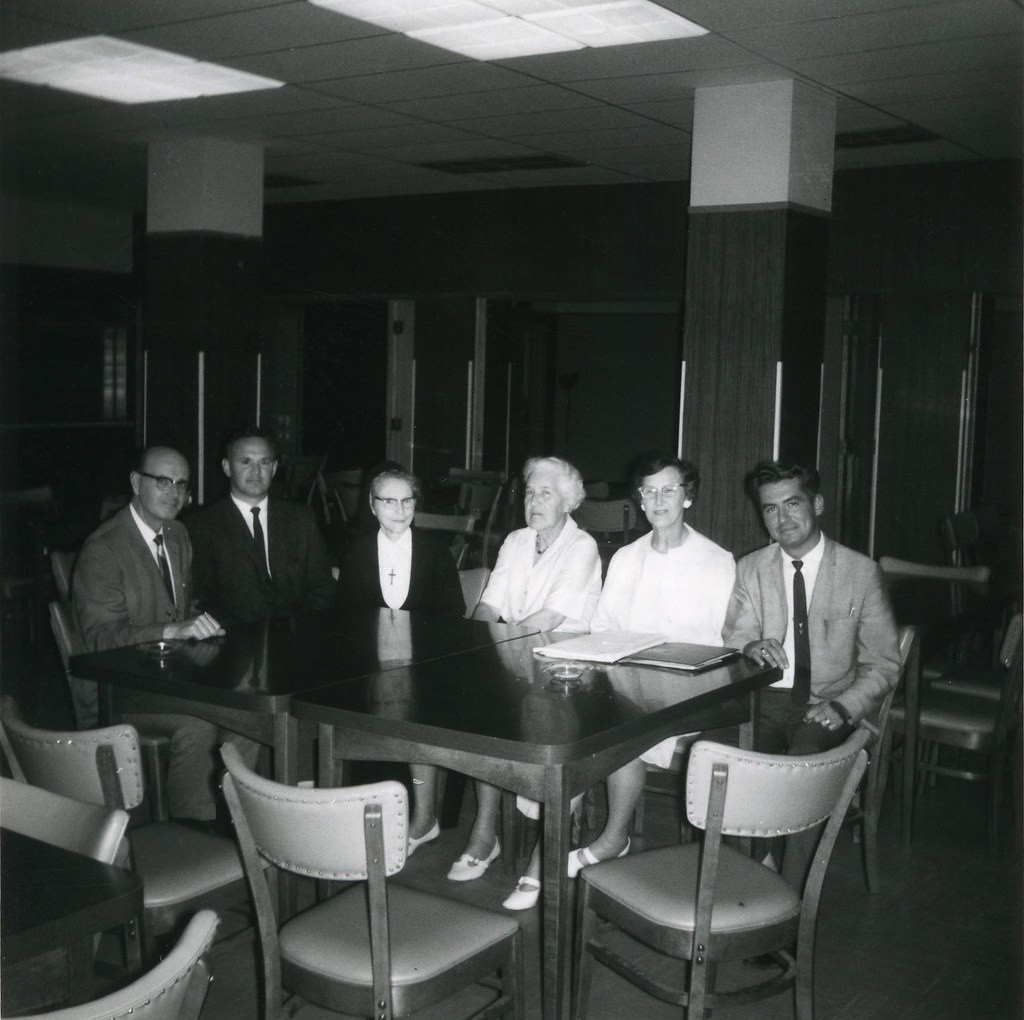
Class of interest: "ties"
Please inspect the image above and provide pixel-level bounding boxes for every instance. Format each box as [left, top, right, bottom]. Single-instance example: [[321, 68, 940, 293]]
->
[[250, 506, 266, 564], [153, 535, 177, 607], [791, 561, 812, 707]]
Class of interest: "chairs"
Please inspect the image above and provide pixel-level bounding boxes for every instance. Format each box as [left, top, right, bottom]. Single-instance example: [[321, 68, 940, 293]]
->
[[0, 472, 1024, 1020]]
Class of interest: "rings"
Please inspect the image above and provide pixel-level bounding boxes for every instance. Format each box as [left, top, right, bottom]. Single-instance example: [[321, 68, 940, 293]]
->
[[825, 718, 830, 726], [760, 648, 766, 654]]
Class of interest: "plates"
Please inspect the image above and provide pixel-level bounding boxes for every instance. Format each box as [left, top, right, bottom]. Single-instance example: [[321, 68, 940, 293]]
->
[[542, 660, 594, 681], [136, 640, 184, 656]]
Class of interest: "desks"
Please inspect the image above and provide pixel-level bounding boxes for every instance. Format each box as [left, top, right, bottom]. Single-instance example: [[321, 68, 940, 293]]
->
[[64, 621, 541, 927], [287, 627, 782, 1020]]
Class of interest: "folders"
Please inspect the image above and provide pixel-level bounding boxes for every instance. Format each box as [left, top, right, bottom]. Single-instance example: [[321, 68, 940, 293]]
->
[[618, 642, 741, 676]]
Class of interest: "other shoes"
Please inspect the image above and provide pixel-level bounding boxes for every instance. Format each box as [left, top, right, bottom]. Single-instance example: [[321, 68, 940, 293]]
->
[[503, 875, 542, 911], [448, 834, 500, 882], [567, 836, 631, 879], [407, 818, 440, 858]]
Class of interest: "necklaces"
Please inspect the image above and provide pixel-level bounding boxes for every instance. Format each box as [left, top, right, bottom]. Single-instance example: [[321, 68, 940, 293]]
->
[[536, 534, 548, 553], [387, 568, 396, 585]]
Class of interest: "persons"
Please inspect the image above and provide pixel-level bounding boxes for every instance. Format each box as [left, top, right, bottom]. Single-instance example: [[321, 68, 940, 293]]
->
[[487, 623, 600, 760], [351, 606, 440, 722], [186, 427, 333, 628], [70, 445, 260, 839], [136, 640, 219, 668], [333, 469, 467, 618], [408, 456, 603, 882], [721, 462, 902, 966], [502, 455, 735, 910]]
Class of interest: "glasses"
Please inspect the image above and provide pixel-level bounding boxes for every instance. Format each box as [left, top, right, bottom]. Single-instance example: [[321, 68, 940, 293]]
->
[[138, 471, 194, 494], [374, 496, 417, 508], [637, 484, 687, 498]]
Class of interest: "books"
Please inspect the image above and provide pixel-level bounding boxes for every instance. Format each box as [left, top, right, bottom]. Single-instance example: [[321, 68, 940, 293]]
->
[[618, 642, 740, 672]]
